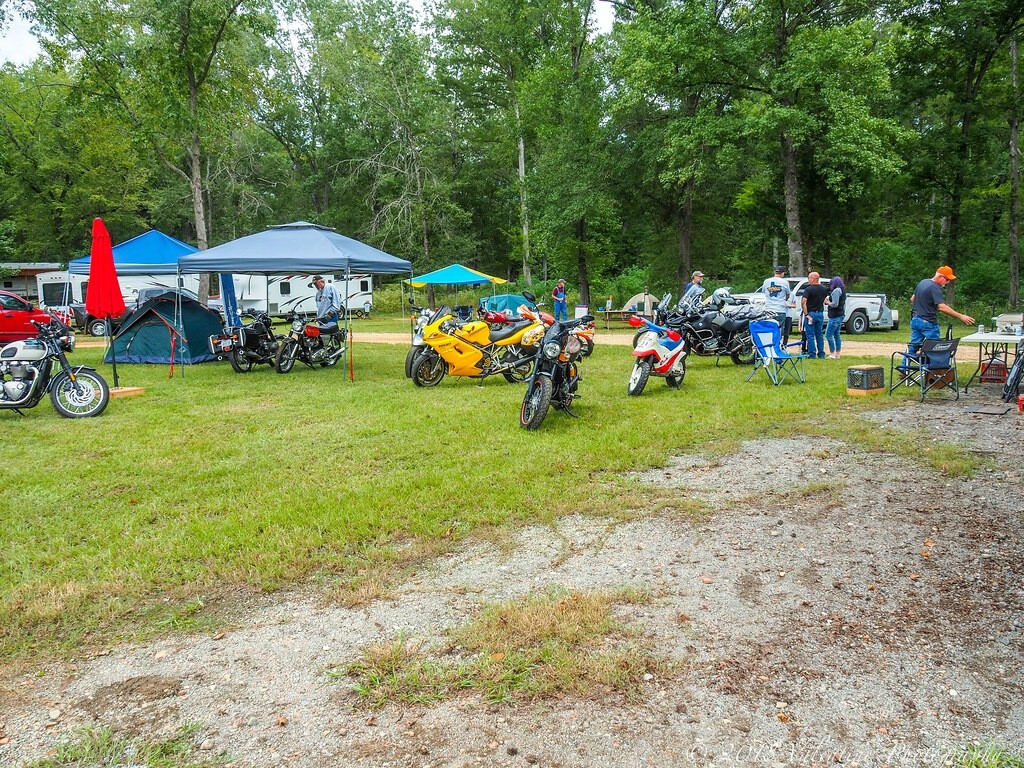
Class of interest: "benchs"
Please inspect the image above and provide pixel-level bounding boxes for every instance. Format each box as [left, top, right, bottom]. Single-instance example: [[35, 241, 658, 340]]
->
[[602, 317, 639, 322]]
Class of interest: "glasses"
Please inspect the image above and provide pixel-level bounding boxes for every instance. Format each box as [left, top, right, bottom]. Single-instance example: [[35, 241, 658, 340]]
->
[[943, 276, 950, 282], [808, 276, 813, 280]]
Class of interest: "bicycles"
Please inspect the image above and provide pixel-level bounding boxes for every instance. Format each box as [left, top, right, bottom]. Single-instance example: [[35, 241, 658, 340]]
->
[[1000, 337, 1024, 404]]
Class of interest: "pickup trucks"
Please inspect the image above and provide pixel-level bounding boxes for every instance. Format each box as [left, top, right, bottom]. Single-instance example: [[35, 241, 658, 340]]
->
[[69, 285, 228, 336], [722, 276, 887, 336]]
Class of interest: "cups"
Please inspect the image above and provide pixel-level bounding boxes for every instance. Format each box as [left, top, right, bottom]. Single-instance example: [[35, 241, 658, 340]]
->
[[1016, 327, 1021, 336], [978, 325, 984, 334], [808, 318, 812, 325], [825, 299, 828, 305]]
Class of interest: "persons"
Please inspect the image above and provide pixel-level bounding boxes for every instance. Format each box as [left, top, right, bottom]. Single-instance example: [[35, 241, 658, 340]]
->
[[762, 266, 796, 350], [552, 279, 567, 321], [824, 276, 846, 359], [899, 266, 975, 378], [685, 271, 705, 307], [312, 275, 341, 326], [798, 272, 828, 358]]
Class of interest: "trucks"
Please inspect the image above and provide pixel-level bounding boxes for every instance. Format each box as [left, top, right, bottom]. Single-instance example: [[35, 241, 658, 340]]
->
[[231, 271, 374, 323], [33, 271, 200, 320]]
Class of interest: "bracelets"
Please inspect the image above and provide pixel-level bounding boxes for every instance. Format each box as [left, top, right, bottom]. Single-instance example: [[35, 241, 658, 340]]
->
[[805, 314, 808, 316]]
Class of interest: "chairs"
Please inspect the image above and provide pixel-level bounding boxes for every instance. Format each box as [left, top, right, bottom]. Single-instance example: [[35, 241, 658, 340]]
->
[[745, 319, 807, 386], [457, 306, 473, 322], [889, 337, 960, 403]]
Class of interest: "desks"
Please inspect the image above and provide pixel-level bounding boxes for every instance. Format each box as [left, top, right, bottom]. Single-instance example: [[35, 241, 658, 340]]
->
[[991, 316, 997, 331], [960, 332, 1024, 394], [603, 311, 638, 330]]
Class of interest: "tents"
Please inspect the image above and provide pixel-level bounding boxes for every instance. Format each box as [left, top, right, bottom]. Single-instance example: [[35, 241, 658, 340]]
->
[[63, 229, 202, 324], [623, 293, 660, 311], [179, 221, 413, 380], [401, 263, 508, 330], [480, 294, 537, 317], [104, 291, 224, 365]]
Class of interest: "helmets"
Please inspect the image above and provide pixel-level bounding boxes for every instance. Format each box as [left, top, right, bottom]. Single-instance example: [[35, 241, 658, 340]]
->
[[713, 288, 730, 305]]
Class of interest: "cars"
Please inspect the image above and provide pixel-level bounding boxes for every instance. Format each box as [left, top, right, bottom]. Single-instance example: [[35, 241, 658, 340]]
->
[[0, 288, 57, 346]]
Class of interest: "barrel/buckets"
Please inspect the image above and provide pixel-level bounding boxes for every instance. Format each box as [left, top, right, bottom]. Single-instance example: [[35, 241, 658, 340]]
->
[[637, 301, 644, 311]]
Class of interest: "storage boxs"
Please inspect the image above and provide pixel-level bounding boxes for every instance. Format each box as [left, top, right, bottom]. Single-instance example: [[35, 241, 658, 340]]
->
[[981, 363, 1007, 384], [1018, 394, 1024, 411], [927, 369, 955, 389], [848, 368, 885, 390], [996, 313, 1023, 335]]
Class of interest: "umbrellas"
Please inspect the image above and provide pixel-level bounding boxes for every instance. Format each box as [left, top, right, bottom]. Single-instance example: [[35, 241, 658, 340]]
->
[[86, 217, 126, 387]]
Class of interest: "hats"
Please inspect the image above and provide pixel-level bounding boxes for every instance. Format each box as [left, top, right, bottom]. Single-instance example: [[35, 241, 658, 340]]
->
[[558, 278, 566, 284], [693, 271, 705, 277], [775, 266, 786, 272], [312, 275, 322, 284], [937, 266, 957, 280]]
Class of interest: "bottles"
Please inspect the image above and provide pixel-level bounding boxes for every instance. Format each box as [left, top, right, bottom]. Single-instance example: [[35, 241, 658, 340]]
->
[[560, 295, 563, 303]]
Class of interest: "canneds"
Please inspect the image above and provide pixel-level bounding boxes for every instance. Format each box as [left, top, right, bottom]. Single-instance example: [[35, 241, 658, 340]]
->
[[825, 299, 828, 305], [1016, 327, 1021, 335], [808, 318, 812, 324], [979, 325, 984, 335]]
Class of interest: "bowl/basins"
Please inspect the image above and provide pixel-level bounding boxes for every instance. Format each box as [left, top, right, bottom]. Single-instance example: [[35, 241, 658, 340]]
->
[[598, 307, 603, 310]]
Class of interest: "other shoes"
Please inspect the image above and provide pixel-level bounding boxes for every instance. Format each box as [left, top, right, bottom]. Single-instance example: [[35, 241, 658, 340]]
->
[[801, 349, 807, 354], [898, 372, 906, 379], [807, 354, 812, 359], [828, 354, 840, 359]]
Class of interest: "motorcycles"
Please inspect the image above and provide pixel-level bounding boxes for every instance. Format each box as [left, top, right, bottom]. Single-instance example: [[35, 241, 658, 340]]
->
[[207, 307, 291, 374], [518, 288, 597, 430], [403, 291, 555, 388], [273, 304, 350, 374], [0, 301, 110, 419], [627, 285, 780, 397]]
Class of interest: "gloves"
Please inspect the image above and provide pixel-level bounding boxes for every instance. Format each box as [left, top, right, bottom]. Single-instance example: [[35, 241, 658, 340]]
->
[[326, 312, 333, 320]]
